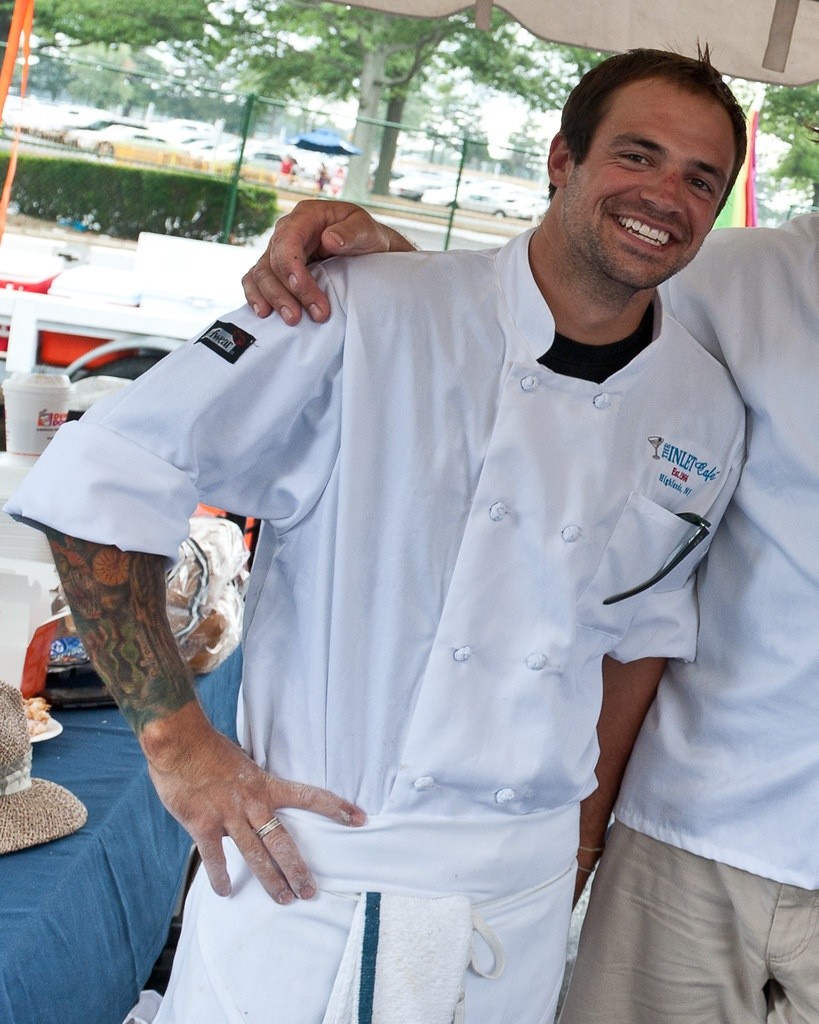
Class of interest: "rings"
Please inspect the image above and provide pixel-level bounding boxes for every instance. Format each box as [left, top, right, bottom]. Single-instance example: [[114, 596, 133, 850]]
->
[[256, 816, 282, 840]]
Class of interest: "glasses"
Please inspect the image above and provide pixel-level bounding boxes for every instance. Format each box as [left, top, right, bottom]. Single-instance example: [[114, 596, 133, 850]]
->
[[602, 511, 712, 604]]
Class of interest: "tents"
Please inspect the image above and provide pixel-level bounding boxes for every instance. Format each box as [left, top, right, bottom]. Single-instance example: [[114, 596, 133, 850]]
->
[[321, 0, 819, 91]]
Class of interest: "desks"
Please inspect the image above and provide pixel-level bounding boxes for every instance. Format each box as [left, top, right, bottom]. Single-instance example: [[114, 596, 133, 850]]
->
[[0, 643, 247, 1024]]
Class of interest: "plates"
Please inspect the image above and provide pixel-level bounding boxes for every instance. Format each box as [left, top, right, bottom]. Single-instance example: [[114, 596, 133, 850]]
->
[[28, 716, 64, 742]]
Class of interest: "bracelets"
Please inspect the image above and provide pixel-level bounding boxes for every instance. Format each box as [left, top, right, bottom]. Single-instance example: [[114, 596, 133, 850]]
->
[[577, 866, 596, 872], [576, 845, 603, 852]]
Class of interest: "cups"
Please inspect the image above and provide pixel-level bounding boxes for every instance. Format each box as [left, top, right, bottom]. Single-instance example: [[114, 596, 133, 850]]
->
[[1, 371, 78, 467]]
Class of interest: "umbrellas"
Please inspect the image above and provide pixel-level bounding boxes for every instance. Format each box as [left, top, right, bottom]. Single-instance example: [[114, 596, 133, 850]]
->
[[285, 129, 362, 157]]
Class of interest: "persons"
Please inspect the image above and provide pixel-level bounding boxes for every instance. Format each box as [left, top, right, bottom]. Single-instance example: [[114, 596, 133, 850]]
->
[[1, 41, 752, 1023], [243, 180, 818, 1020], [276, 153, 346, 194]]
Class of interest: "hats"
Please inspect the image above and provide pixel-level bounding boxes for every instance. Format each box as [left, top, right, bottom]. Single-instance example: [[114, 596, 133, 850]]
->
[[2, 683, 88, 860]]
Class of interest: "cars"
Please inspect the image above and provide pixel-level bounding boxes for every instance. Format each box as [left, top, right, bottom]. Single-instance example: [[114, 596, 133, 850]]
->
[[5, 93, 553, 224]]
